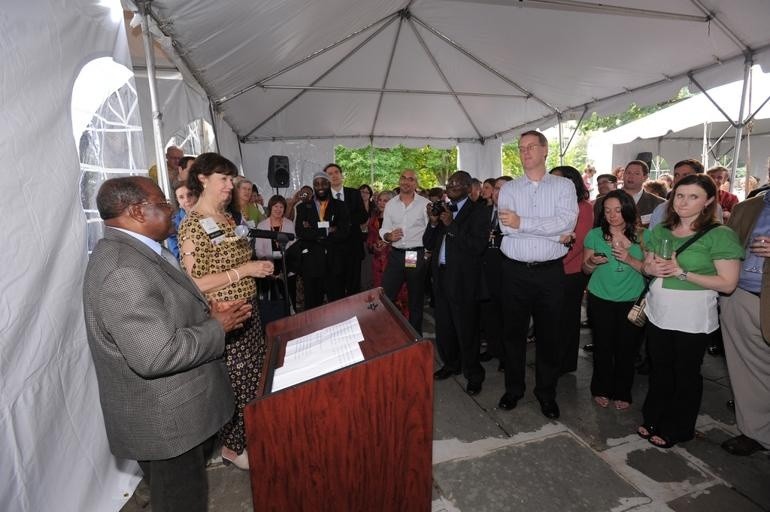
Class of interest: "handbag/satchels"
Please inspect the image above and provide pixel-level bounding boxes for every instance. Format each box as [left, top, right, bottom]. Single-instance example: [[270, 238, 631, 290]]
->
[[627, 289, 647, 327]]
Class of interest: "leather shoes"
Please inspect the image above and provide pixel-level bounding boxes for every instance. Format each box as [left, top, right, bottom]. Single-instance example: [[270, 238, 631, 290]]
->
[[584, 342, 593, 351]]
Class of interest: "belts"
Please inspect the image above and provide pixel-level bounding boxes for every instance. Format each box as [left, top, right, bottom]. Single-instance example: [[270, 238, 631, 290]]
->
[[522, 260, 544, 269]]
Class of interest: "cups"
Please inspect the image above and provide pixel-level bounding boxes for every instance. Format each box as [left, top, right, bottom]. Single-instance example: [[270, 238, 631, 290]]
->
[[656, 239, 673, 260]]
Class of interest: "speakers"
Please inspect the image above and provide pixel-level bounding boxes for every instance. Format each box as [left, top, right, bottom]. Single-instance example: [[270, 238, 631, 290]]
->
[[635, 152, 652, 173], [268, 155, 290, 188]]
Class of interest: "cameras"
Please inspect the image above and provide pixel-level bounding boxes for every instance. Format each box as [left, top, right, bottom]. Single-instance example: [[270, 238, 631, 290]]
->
[[300, 192, 308, 199], [430, 200, 459, 217]]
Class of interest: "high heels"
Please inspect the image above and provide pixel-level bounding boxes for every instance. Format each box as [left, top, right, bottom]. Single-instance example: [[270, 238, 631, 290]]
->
[[222, 449, 249, 471]]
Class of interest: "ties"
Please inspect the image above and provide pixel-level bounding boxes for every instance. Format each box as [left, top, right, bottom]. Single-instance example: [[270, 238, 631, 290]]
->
[[336, 193, 341, 200], [161, 246, 182, 272]]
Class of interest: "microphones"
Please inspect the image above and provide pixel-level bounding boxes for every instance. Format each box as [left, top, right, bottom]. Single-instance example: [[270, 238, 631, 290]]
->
[[234, 224, 295, 241]]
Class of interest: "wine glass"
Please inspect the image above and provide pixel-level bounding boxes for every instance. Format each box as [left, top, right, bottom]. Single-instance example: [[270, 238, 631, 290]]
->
[[745, 232, 765, 276], [497, 203, 513, 236], [611, 240, 627, 273]]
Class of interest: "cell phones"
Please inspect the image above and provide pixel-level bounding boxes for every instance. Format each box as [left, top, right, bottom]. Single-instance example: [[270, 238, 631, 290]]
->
[[594, 253, 608, 258]]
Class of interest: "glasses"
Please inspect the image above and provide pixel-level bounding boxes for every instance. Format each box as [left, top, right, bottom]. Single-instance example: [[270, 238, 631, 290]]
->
[[519, 144, 545, 151]]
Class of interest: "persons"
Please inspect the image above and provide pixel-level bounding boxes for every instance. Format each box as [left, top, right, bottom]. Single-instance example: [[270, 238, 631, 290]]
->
[[149, 145, 198, 258], [498, 129, 579, 422], [636, 173, 748, 449], [718, 157, 770, 455], [366, 167, 535, 396], [234, 163, 369, 331], [80, 175, 253, 512], [549, 160, 769, 408], [174, 150, 274, 471]]
